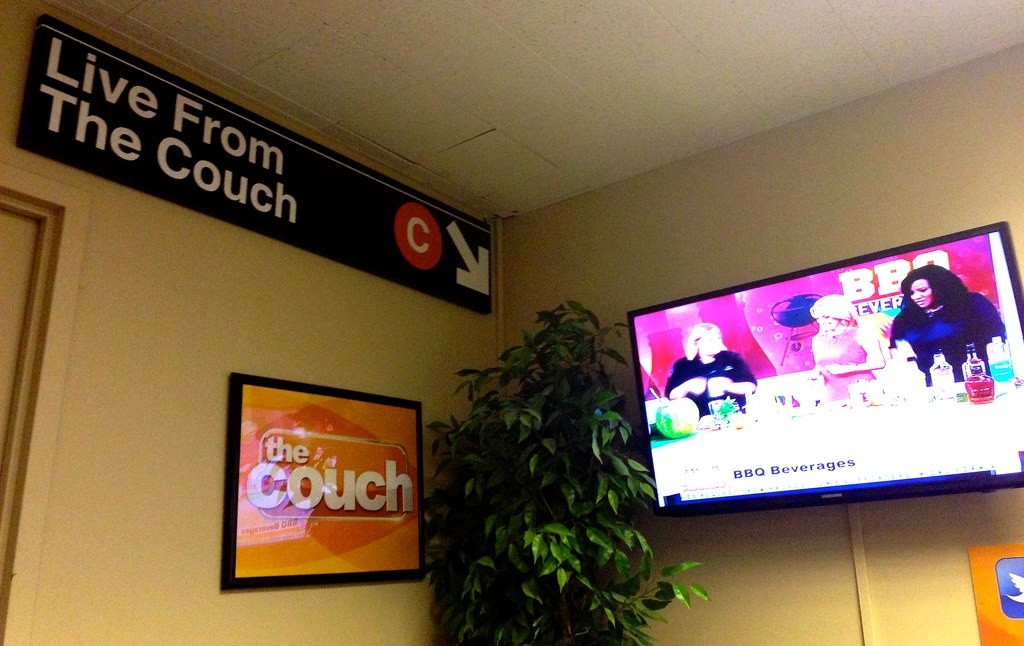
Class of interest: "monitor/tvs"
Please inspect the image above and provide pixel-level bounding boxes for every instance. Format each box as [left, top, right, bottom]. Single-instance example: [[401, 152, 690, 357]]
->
[[628, 220, 1024, 517]]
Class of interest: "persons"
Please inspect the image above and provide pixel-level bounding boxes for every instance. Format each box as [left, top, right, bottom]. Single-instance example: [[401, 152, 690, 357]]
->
[[663, 323, 757, 421], [807, 294, 885, 403], [888, 265, 1008, 387]]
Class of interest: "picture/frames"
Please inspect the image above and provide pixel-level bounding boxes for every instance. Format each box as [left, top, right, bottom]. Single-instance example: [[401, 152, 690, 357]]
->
[[221, 371, 426, 592]]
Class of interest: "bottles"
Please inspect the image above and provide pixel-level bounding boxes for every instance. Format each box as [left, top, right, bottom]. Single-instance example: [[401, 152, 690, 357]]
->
[[929, 349, 956, 403], [989, 336, 1015, 387], [962, 343, 986, 379], [964, 367, 995, 404]]
